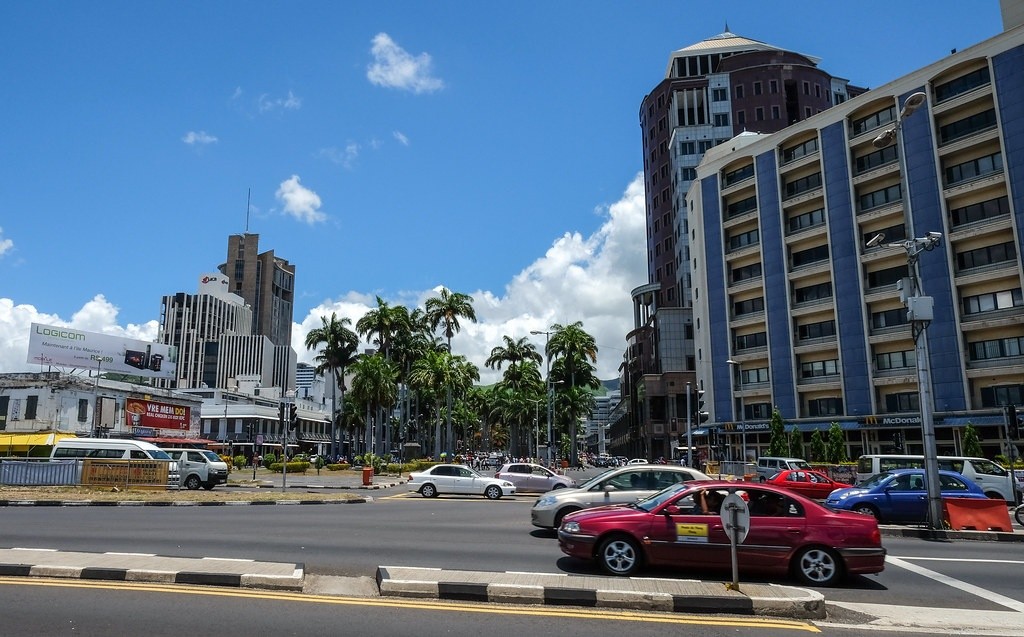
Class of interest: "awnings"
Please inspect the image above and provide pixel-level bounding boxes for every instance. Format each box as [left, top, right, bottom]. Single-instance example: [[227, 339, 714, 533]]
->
[[0, 433, 78, 446]]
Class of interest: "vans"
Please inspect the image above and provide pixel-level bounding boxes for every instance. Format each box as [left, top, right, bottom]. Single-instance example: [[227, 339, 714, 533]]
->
[[49, 439, 228, 491], [856, 454, 1023, 505], [756, 457, 812, 483]]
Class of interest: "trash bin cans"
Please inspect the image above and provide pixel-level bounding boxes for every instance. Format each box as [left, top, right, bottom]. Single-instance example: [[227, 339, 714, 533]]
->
[[562, 461, 568, 468], [363, 467, 374, 485]]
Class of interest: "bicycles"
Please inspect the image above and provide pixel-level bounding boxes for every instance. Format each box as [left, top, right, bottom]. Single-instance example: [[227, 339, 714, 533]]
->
[[1015, 504, 1024, 526]]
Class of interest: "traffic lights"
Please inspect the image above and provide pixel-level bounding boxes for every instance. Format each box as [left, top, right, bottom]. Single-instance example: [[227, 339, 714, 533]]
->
[[1009, 405, 1024, 440], [694, 389, 709, 430], [288, 402, 298, 432]]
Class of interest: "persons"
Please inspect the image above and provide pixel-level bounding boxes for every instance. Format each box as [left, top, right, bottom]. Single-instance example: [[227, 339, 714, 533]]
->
[[496, 456, 543, 466], [474, 455, 490, 471], [613, 457, 628, 468], [337, 454, 347, 464], [681, 455, 707, 472], [893, 476, 909, 488], [577, 455, 585, 471], [630, 474, 642, 488], [248, 454, 264, 470], [692, 491, 720, 515]]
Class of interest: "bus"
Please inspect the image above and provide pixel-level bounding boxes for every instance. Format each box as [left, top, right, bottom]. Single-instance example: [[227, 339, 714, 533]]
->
[[207, 443, 299, 460]]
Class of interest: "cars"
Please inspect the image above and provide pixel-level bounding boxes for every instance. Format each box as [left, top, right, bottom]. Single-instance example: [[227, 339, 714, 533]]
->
[[293, 454, 332, 465], [408, 463, 572, 501], [531, 464, 751, 534], [556, 479, 886, 587], [597, 453, 649, 465], [825, 468, 988, 524], [765, 470, 853, 499]]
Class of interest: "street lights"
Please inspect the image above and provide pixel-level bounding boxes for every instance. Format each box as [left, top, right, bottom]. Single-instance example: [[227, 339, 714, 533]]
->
[[223, 385, 237, 453], [550, 380, 564, 462], [872, 92, 945, 527], [726, 359, 746, 475], [530, 330, 552, 466], [89, 358, 102, 438]]
